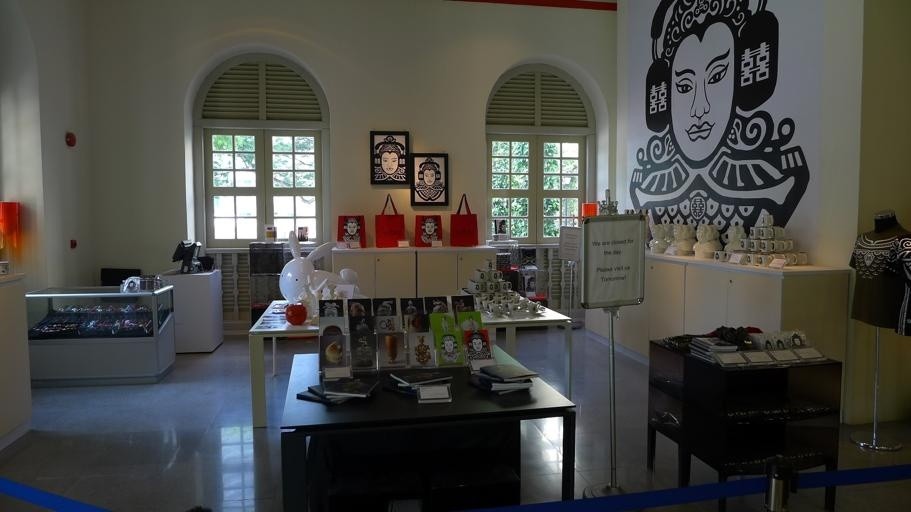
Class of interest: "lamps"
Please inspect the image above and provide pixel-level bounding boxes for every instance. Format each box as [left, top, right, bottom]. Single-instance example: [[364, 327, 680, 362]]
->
[[582, 202, 597, 218], [0, 201, 20, 248]]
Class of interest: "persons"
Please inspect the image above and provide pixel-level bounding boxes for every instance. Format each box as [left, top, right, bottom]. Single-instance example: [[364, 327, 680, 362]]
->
[[415, 157, 446, 201], [496, 219, 507, 234], [441, 333, 462, 362], [764, 333, 806, 351], [843, 209, 911, 335], [467, 333, 491, 359], [373, 136, 408, 183], [629, 1, 796, 241], [342, 218, 361, 242], [524, 275, 537, 293], [419, 218, 439, 245]]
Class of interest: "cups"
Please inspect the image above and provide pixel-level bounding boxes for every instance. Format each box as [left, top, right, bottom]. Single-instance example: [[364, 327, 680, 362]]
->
[[713, 214, 808, 266], [457, 257, 541, 319]]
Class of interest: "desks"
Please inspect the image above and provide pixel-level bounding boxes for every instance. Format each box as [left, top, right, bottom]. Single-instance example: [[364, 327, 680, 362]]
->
[[248, 296, 574, 428], [646, 334, 842, 512], [279, 344, 576, 512]]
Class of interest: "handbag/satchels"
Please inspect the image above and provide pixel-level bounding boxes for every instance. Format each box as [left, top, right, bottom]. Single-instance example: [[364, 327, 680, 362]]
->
[[376, 194, 404, 248], [450, 194, 478, 247]]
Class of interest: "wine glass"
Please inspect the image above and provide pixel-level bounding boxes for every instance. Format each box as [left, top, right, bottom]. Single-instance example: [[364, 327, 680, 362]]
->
[[384, 335, 400, 365]]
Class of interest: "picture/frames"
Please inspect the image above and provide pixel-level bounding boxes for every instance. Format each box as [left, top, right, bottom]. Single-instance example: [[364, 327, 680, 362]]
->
[[409, 153, 449, 207], [370, 131, 409, 184]]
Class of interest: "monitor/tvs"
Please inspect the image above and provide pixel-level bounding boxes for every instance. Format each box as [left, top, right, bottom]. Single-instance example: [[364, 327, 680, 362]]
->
[[172, 240, 192, 263]]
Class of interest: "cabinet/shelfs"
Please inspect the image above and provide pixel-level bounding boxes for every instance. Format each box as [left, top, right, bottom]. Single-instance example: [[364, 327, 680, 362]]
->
[[333, 253, 417, 299], [584, 248, 851, 425], [417, 252, 497, 299]]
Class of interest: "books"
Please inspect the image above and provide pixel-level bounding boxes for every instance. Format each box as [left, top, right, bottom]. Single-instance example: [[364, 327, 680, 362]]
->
[[296, 362, 541, 404], [689, 335, 741, 363]]
